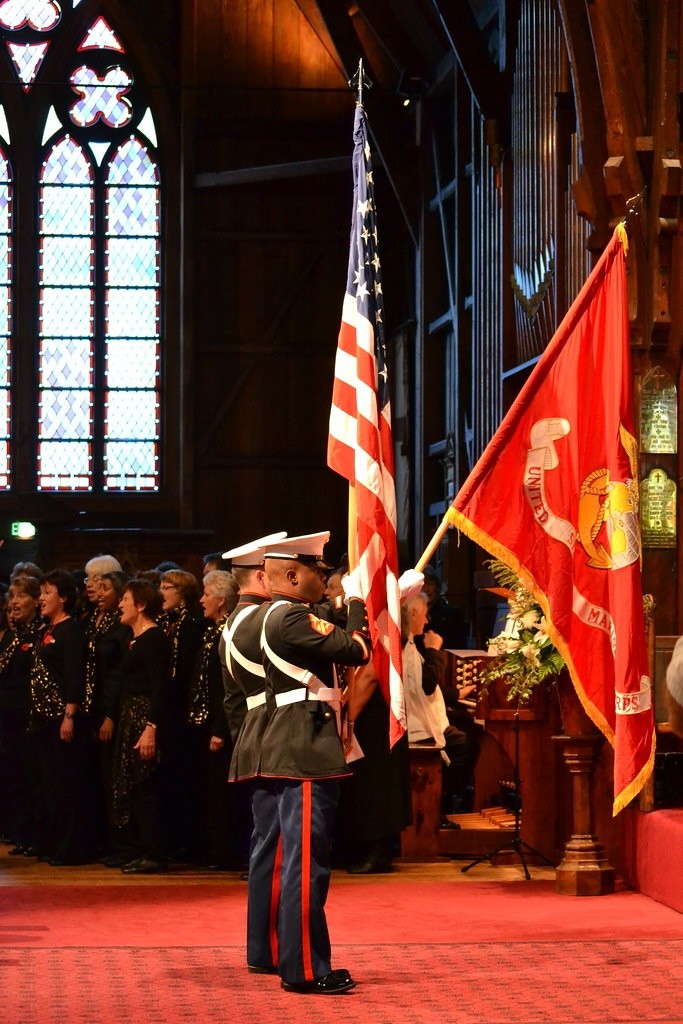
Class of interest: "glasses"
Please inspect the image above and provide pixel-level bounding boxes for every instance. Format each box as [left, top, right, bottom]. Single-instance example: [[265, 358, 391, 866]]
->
[[84, 576, 102, 584], [160, 585, 175, 591]]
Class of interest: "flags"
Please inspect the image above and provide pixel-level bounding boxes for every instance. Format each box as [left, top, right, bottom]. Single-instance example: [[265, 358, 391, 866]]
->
[[326, 106, 411, 754], [440, 222, 658, 818]]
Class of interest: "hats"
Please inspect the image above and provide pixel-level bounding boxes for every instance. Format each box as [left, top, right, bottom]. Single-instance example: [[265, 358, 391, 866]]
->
[[221, 532, 287, 568], [256, 530, 334, 571]]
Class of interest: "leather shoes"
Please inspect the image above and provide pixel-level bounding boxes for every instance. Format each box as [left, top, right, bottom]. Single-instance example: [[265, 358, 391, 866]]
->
[[241, 873, 249, 880], [8, 845, 38, 857], [280, 968, 356, 995], [196, 864, 219, 871], [248, 964, 276, 972], [439, 822, 459, 829], [38, 852, 68, 866], [121, 857, 159, 874], [346, 861, 392, 875]]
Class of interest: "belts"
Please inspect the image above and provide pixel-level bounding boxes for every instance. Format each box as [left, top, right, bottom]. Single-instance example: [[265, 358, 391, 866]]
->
[[245, 690, 268, 711], [275, 684, 340, 708]]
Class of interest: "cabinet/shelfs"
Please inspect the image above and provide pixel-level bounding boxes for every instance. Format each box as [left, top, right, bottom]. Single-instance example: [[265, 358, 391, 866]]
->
[[175, 84, 414, 623], [417, 0, 683, 638]]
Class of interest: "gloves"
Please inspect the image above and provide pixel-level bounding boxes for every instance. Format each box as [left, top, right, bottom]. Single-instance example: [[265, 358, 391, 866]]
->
[[340, 563, 366, 602], [398, 569, 425, 599]]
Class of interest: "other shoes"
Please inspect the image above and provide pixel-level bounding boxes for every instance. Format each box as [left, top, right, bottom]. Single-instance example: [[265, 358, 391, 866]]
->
[[0, 837, 15, 845], [96, 855, 127, 867]]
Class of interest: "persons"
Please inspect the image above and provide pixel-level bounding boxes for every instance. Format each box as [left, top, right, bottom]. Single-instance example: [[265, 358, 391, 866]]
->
[[1, 554, 448, 874], [219, 531, 426, 975], [254, 531, 374, 994]]
